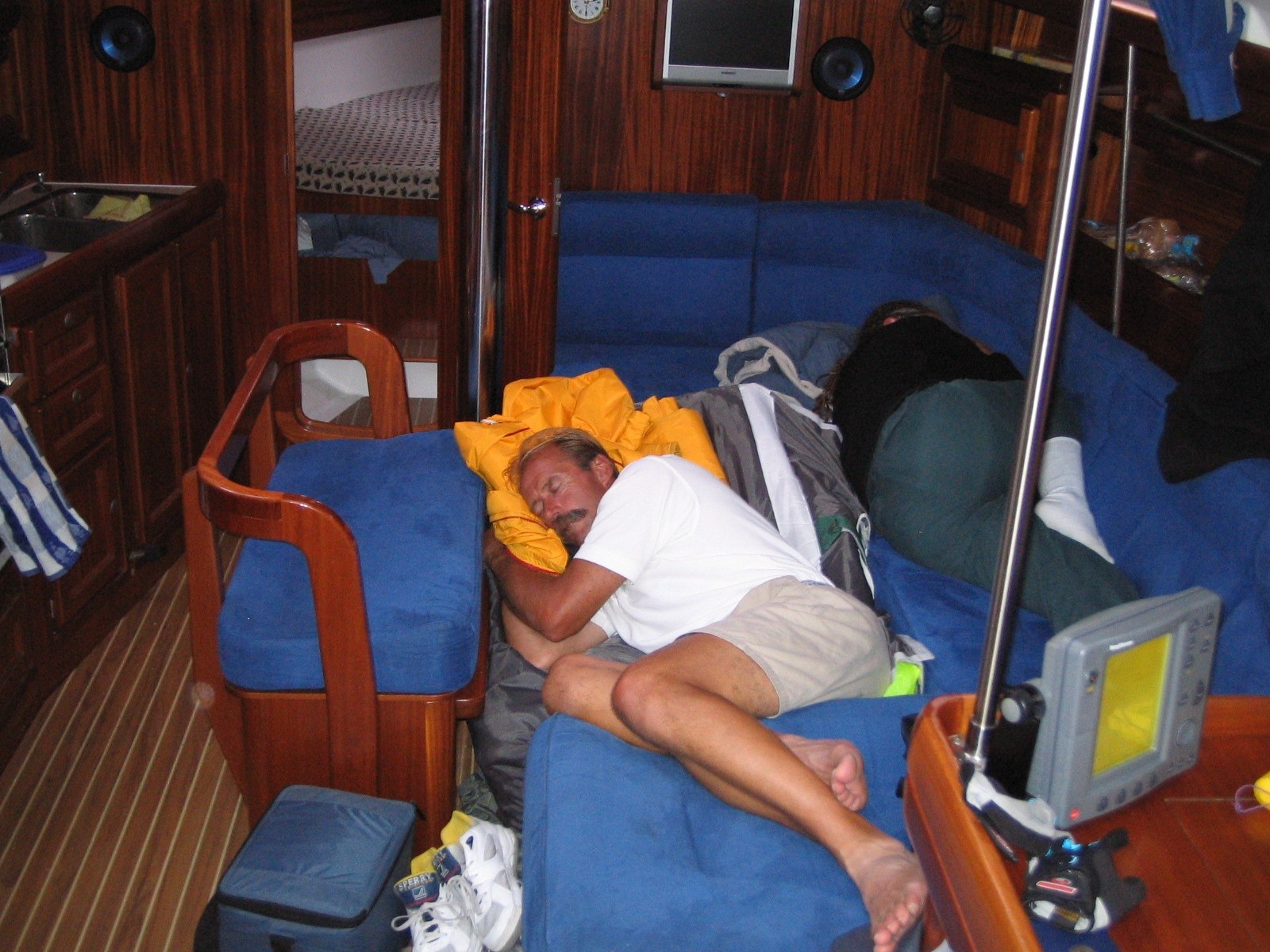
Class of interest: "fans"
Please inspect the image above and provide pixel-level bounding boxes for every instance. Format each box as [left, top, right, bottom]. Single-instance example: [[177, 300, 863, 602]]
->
[[897, 0, 964, 46]]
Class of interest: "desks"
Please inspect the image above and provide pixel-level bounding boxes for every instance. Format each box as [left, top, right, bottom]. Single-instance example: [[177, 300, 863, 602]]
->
[[910, 680, 1270, 952]]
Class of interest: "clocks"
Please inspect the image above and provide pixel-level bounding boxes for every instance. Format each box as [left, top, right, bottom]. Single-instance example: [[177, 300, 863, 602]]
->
[[569, 0, 610, 25]]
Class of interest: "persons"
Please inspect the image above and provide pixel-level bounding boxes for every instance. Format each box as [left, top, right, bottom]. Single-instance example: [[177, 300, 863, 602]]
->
[[815, 299, 1139, 633], [481, 427, 930, 952]]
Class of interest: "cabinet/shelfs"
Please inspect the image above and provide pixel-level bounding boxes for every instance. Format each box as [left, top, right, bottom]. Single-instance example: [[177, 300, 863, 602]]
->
[[0, 207, 230, 772], [930, 0, 1270, 352]]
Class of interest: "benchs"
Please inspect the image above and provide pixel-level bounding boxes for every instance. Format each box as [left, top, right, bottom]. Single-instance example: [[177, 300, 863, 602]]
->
[[189, 317, 494, 845]]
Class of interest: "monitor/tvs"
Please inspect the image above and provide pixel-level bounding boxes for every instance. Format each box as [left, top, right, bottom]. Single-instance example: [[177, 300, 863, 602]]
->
[[663, 0, 800, 90], [1027, 587, 1220, 829]]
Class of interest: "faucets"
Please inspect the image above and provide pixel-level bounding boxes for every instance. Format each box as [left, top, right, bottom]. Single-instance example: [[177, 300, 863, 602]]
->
[[1, 170, 45, 205]]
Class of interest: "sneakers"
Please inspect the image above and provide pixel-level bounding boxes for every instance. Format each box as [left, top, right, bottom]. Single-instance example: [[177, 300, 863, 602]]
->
[[439, 812, 523, 952], [390, 847, 483, 952]]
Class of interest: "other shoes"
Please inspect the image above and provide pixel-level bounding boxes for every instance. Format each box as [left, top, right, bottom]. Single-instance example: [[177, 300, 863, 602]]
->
[[967, 772, 1076, 863]]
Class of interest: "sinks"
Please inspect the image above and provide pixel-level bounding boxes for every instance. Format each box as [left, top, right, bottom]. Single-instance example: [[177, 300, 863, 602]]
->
[[0, 210, 124, 253], [25, 187, 179, 228]]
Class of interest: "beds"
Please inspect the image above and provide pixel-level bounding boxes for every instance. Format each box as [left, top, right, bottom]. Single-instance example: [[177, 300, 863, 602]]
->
[[474, 180, 1270, 952]]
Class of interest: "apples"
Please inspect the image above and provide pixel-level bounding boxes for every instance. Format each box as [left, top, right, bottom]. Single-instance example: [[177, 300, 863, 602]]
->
[[1125, 217, 1200, 292]]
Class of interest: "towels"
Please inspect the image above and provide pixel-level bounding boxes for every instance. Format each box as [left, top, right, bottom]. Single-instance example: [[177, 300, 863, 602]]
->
[[1, 395, 92, 582]]
